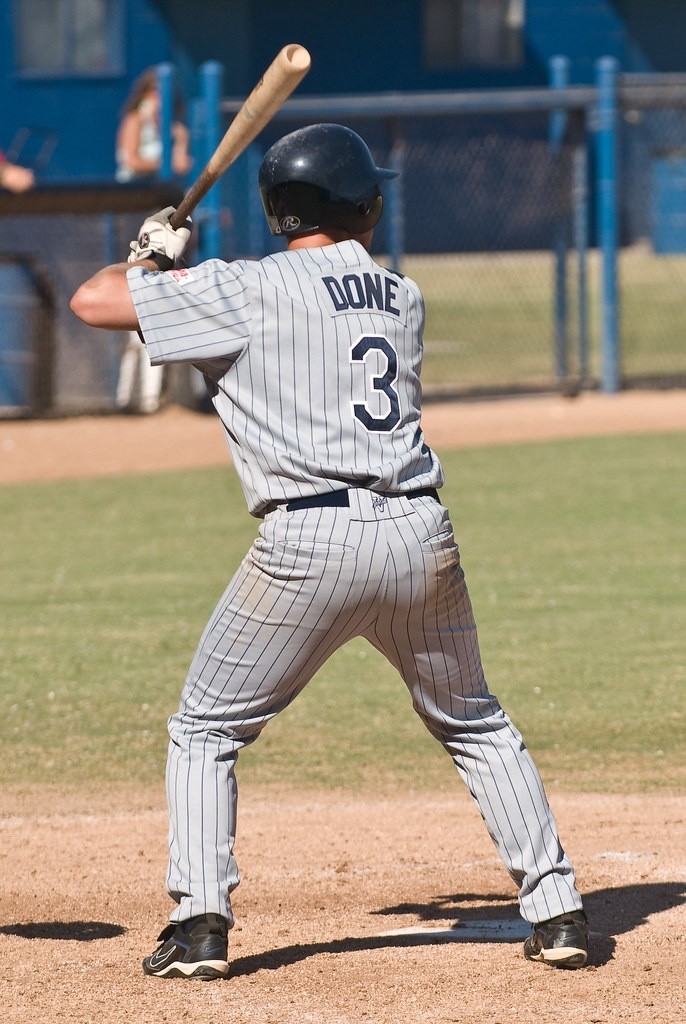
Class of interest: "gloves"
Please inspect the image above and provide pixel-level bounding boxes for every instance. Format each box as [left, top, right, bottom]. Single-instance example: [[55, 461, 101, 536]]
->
[[128, 204, 196, 265]]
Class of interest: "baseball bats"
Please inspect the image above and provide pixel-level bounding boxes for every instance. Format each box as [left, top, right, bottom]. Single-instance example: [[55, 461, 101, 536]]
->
[[172, 41, 314, 225]]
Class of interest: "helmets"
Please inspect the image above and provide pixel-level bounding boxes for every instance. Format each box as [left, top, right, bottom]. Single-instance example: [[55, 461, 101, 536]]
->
[[258, 123, 401, 237]]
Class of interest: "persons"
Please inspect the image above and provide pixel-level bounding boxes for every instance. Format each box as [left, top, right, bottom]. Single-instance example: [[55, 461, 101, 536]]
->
[[0, 155, 36, 194], [114, 59, 195, 273], [70, 123, 589, 982]]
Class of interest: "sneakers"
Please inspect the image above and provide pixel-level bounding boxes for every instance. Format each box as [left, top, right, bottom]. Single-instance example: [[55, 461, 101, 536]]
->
[[525, 914, 589, 969], [142, 914, 231, 982]]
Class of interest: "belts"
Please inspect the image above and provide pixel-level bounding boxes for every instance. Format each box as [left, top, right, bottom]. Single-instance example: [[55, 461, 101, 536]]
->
[[288, 488, 356, 510]]
